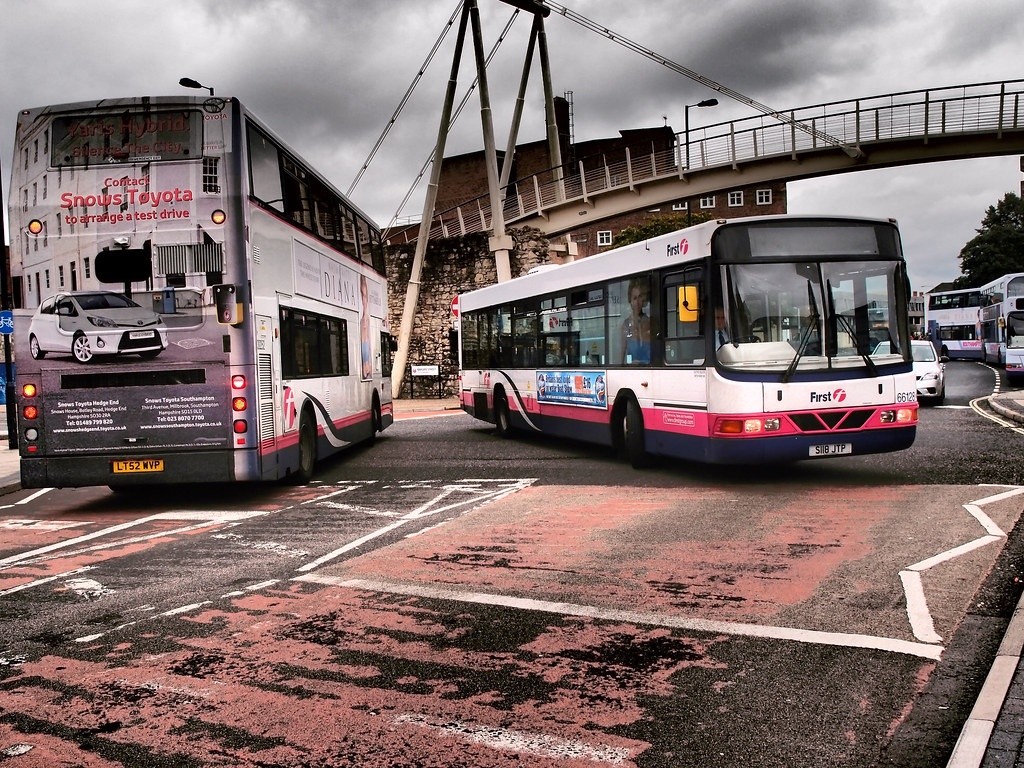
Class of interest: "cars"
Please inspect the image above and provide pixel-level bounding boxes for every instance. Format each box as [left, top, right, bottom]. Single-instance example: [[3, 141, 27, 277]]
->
[[28, 291, 170, 365], [872, 340, 950, 403]]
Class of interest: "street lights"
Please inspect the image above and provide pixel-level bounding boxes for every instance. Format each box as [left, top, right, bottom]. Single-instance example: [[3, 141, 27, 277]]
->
[[179, 78, 214, 96], [685, 99, 719, 227]]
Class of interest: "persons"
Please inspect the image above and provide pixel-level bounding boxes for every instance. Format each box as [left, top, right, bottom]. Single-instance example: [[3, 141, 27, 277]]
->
[[910, 331, 915, 340], [538, 374, 545, 389], [976, 309, 981, 340], [479, 371, 490, 388], [692, 306, 737, 362], [360, 275, 372, 380], [546, 340, 568, 366], [918, 333, 929, 340], [621, 276, 651, 365]]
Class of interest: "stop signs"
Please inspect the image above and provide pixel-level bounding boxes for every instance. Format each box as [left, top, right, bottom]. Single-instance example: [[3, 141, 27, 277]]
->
[[451, 295, 458, 318]]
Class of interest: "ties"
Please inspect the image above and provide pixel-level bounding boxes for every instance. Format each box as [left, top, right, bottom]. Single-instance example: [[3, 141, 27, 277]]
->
[[718, 330, 725, 346]]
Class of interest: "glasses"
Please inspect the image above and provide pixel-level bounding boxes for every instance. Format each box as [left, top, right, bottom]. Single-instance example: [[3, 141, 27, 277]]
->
[[715, 316, 725, 321]]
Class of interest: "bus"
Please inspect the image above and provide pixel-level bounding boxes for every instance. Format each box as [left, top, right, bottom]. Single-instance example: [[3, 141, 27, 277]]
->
[[459, 214, 919, 469], [924, 273, 1024, 379], [8, 97, 392, 494]]
